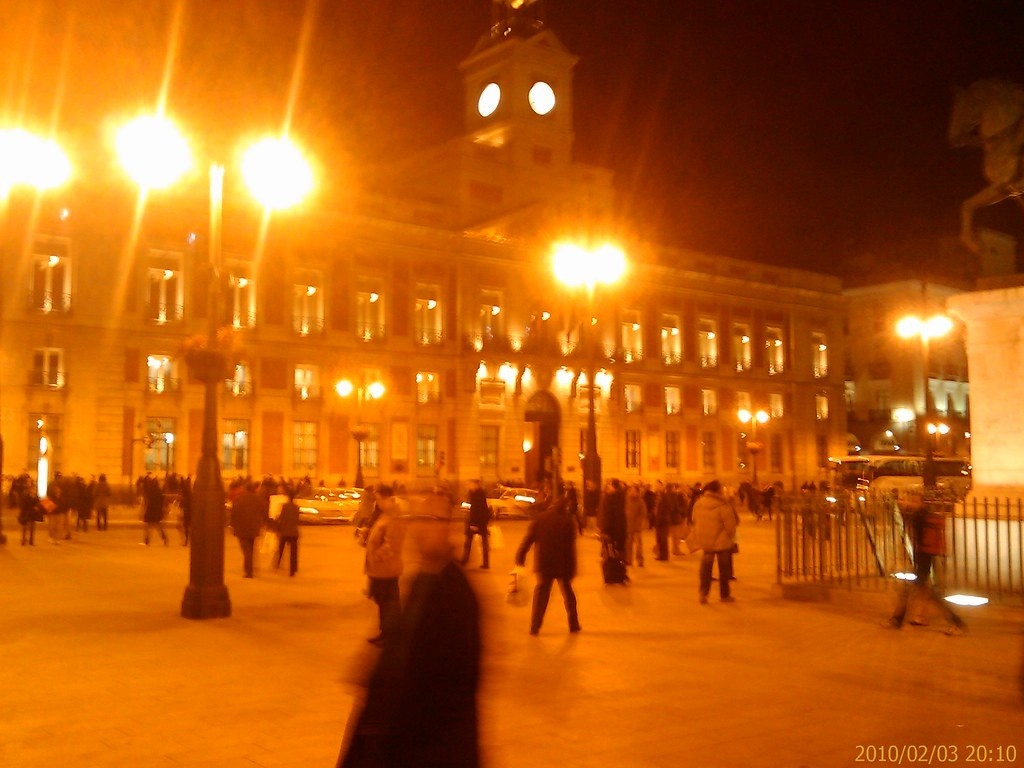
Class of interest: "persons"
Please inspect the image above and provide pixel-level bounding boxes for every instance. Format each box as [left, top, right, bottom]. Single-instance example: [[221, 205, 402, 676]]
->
[[335, 522, 481, 768], [352, 471, 587, 650], [8, 471, 200, 548], [596, 478, 819, 606], [225, 473, 347, 577], [879, 460, 967, 630]]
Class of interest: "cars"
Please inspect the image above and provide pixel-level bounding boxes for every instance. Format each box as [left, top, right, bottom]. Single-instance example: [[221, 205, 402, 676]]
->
[[460, 486, 540, 522], [295, 488, 363, 525], [847, 433, 862, 456], [873, 431, 900, 454]]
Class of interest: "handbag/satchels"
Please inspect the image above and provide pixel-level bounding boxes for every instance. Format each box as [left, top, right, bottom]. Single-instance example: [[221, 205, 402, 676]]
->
[[505, 564, 536, 608], [40, 497, 57, 513]]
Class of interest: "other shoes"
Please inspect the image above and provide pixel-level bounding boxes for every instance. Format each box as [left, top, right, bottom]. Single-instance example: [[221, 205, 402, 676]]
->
[[722, 597, 734, 603], [945, 623, 969, 636], [479, 564, 488, 569], [529, 628, 539, 634], [570, 626, 581, 634], [701, 597, 710, 604], [368, 634, 388, 645], [908, 616, 929, 626], [879, 620, 900, 629]]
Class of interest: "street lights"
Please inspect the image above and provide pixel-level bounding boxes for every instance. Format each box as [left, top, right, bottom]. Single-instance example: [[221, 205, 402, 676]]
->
[[738, 408, 770, 485], [550, 237, 632, 518], [118, 107, 321, 624], [893, 313, 953, 489], [336, 375, 387, 489]]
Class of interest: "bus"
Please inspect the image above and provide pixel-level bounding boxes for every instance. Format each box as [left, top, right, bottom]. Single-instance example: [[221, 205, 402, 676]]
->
[[832, 456, 971, 511]]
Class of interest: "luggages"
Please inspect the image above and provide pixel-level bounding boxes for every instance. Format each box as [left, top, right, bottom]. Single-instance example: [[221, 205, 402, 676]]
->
[[603, 539, 625, 583]]
